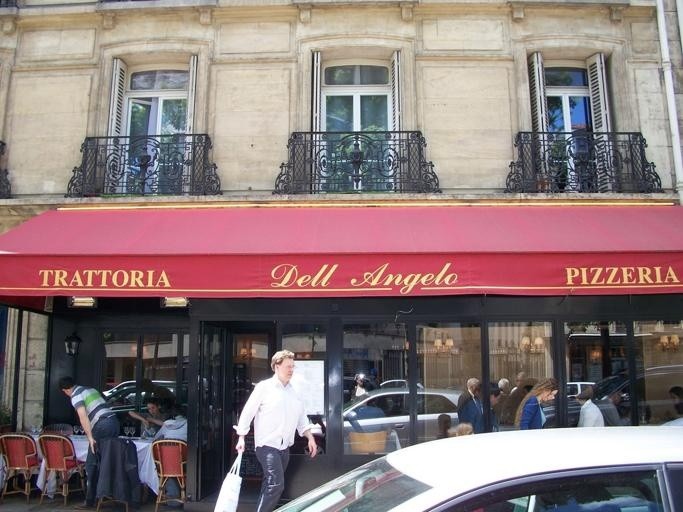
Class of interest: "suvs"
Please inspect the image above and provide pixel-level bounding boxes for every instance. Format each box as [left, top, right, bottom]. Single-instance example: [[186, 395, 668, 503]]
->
[[540, 362, 681, 425]]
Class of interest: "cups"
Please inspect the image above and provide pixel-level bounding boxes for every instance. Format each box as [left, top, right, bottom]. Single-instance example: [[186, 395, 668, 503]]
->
[[30, 425, 36, 433], [36, 425, 41, 434], [140, 424, 145, 439]]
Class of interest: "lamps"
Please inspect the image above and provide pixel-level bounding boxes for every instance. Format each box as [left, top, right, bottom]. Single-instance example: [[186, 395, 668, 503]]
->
[[534, 337, 543, 352], [521, 337, 531, 349], [444, 338, 454, 350], [669, 334, 680, 352], [660, 336, 670, 352], [159, 296, 192, 312], [62, 331, 83, 361], [433, 338, 443, 351], [66, 296, 97, 309]]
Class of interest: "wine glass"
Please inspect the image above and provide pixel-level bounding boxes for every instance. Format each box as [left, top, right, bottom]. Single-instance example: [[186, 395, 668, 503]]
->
[[129, 426, 135, 440], [73, 425, 79, 439], [123, 426, 129, 439], [79, 425, 84, 439]]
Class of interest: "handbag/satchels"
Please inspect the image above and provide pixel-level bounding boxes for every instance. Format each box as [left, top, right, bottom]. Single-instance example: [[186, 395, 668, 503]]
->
[[213, 468, 243, 512]]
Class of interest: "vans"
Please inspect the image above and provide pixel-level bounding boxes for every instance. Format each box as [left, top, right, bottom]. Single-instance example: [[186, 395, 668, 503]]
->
[[103, 381, 176, 397]]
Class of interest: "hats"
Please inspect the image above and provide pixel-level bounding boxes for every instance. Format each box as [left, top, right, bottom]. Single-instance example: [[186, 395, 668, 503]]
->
[[354, 374, 366, 381]]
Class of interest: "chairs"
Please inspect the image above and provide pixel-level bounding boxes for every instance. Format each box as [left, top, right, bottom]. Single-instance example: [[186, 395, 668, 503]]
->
[[348, 431, 387, 455], [0, 425, 187, 511]]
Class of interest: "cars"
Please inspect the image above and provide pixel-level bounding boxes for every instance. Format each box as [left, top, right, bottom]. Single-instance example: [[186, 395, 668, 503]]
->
[[343, 375, 380, 401], [304, 387, 465, 456], [105, 386, 174, 402], [272, 425, 681, 512], [567, 382, 597, 398], [379, 379, 424, 389]]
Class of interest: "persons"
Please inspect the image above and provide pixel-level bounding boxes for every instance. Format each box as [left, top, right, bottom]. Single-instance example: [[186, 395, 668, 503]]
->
[[436, 414, 451, 440], [456, 383, 501, 430], [496, 377, 511, 423], [57, 374, 119, 511], [446, 420, 475, 438], [456, 378, 482, 421], [593, 388, 632, 427], [503, 371, 528, 424], [348, 372, 376, 400], [572, 383, 605, 427], [232, 349, 317, 511], [514, 377, 560, 428], [659, 386, 683, 425], [382, 395, 394, 413], [128, 398, 173, 438], [150, 405, 187, 439]]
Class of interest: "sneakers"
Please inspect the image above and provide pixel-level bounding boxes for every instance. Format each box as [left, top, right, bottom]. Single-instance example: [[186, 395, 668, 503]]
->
[[75, 499, 95, 510]]
